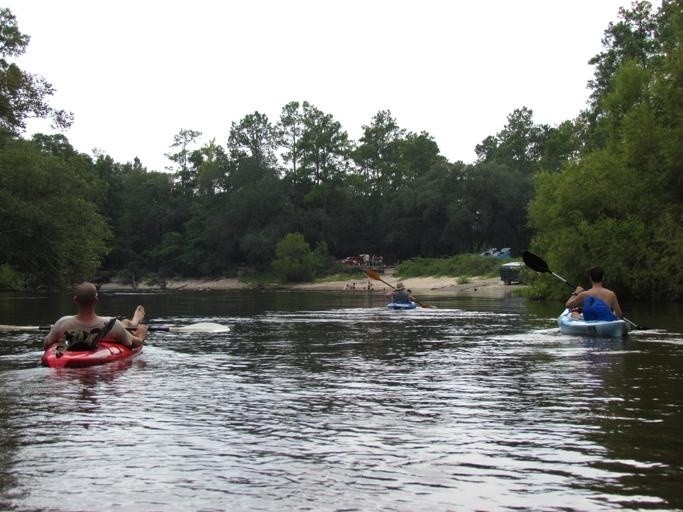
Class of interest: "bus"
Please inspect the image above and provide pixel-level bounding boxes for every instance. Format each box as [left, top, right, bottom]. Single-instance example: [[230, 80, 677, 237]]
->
[[499, 262, 525, 285]]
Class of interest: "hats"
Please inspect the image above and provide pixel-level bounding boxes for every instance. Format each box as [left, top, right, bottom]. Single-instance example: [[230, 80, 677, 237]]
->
[[392, 282, 406, 292]]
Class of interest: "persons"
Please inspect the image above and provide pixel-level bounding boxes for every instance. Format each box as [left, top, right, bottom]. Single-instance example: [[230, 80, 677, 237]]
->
[[386, 282, 416, 303], [565, 268, 623, 322], [40, 281, 148, 347], [342, 253, 383, 291]]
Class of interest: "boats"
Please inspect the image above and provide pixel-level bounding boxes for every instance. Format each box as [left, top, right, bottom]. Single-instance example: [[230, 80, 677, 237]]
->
[[387, 300, 416, 310], [558, 307, 629, 338], [42, 339, 143, 368]]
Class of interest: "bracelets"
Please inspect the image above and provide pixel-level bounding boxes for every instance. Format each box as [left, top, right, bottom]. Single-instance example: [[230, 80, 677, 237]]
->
[[572, 292, 578, 296]]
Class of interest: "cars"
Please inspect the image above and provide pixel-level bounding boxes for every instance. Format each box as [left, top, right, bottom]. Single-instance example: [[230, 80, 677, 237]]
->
[[336, 257, 360, 265]]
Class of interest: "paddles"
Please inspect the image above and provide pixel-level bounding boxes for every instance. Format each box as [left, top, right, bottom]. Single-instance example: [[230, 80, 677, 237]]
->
[[0, 322, 230, 333], [364, 270, 429, 307], [521, 251, 650, 330]]
